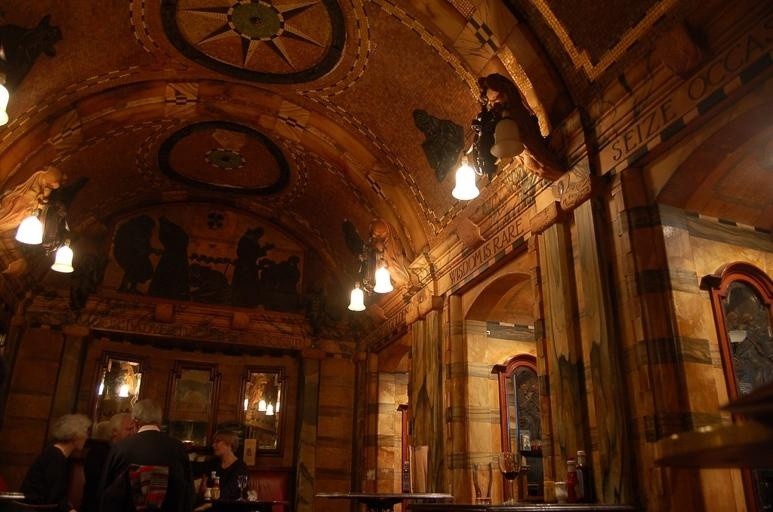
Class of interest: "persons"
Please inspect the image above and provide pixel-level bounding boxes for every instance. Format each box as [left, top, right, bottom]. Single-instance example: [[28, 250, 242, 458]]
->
[[189, 430, 250, 511], [79, 419, 113, 512], [96, 412, 133, 512], [259, 254, 301, 314], [229, 227, 278, 307], [16, 412, 93, 512], [106, 398, 196, 512]]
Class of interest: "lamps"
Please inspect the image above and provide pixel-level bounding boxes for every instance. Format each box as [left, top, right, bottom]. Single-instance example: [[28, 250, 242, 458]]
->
[[347, 258, 394, 312], [14, 197, 76, 274], [728, 329, 749, 352], [450, 73, 527, 201], [96, 368, 129, 399], [255, 388, 277, 418]]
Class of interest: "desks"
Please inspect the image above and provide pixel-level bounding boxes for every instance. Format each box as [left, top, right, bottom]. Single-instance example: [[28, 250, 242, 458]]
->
[[403, 501, 635, 511], [313, 494, 452, 512]]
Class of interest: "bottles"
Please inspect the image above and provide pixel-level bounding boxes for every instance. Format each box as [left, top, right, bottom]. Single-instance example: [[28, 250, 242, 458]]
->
[[566, 449, 588, 502], [542, 479, 565, 503], [402, 459, 410, 493], [204, 470, 220, 501]]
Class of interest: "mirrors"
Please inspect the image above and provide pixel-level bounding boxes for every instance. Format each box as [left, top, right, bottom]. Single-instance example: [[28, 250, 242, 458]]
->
[[84, 349, 146, 425], [234, 364, 288, 459], [161, 358, 221, 453]]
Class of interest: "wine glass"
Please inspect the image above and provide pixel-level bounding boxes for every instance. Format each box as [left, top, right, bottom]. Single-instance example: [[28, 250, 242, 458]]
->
[[235, 474, 248, 502], [497, 451, 522, 505]]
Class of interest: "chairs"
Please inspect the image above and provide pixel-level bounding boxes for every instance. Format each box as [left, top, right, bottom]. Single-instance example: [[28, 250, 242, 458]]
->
[[0, 474, 58, 511], [209, 498, 280, 512]]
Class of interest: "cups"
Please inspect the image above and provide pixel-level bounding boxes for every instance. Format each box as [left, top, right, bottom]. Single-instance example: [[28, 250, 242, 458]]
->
[[471, 460, 493, 505]]
